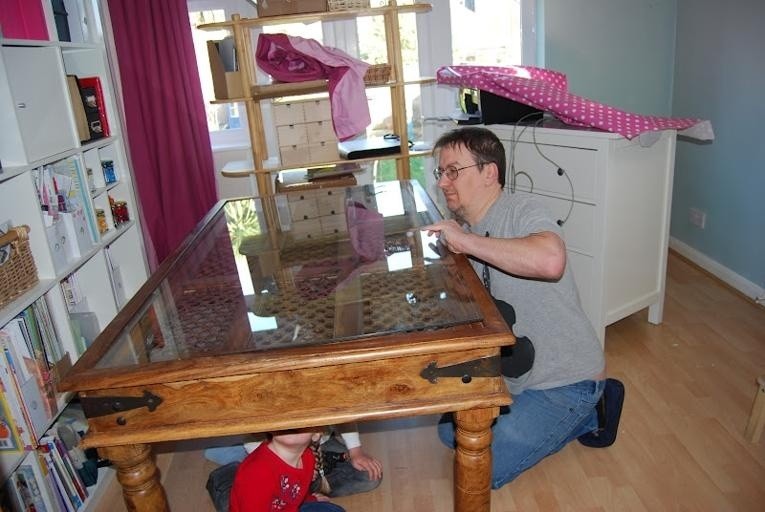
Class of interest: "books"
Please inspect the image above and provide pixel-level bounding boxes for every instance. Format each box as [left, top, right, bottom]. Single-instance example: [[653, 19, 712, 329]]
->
[[0, 295, 99, 511], [32, 154, 101, 245]]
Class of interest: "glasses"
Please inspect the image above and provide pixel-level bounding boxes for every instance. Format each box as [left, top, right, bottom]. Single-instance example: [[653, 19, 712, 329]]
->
[[434, 162, 488, 180]]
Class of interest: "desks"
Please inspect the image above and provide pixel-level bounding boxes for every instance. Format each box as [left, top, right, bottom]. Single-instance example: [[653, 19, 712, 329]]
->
[[57, 180, 516, 512]]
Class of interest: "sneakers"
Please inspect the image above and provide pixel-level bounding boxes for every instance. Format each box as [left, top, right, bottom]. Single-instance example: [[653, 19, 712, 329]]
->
[[577, 379, 624, 448]]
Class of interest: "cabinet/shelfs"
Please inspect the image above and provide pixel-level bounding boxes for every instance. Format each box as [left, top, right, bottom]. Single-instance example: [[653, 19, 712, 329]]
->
[[269, 92, 340, 168], [0, 0, 160, 512], [424, 114, 677, 350], [286, 188, 349, 245], [197, 0, 438, 196]]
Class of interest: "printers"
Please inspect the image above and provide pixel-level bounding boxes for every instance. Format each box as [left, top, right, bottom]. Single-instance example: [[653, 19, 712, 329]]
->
[[451, 86, 546, 124]]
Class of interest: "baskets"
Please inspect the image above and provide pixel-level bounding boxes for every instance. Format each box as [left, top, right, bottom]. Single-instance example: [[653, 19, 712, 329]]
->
[[365, 63, 392, 84], [0, 226, 40, 310]]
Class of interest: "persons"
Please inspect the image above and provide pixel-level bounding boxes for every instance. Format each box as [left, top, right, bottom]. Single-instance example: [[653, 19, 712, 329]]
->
[[203, 418, 385, 497], [418, 127, 627, 491], [228, 424, 347, 511]]
